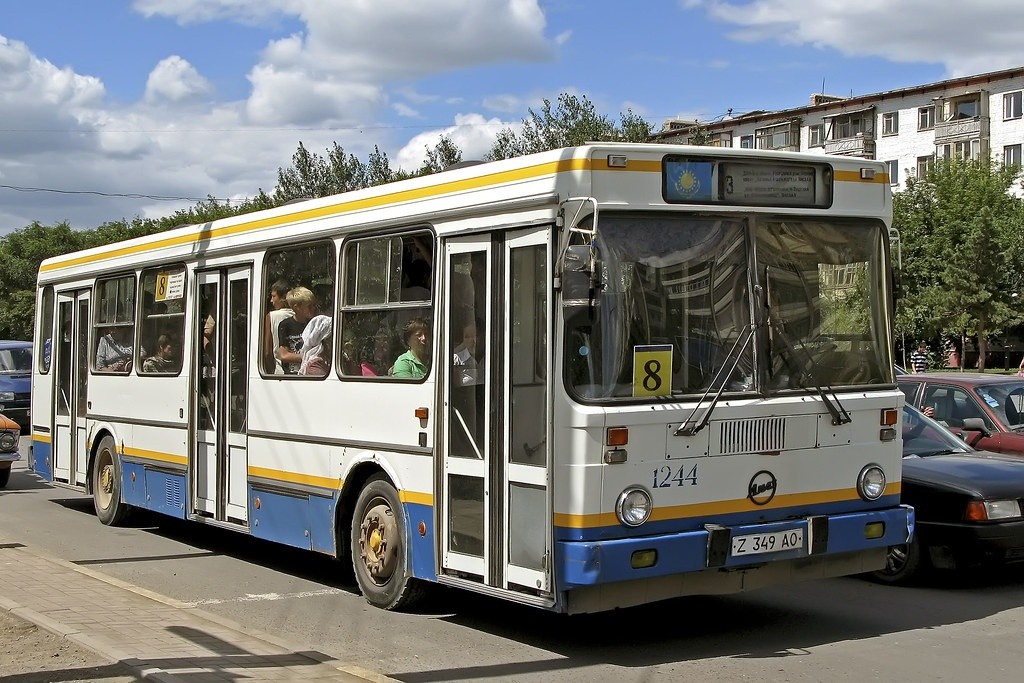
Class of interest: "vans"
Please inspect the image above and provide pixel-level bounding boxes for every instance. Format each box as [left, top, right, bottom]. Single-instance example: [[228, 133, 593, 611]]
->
[[0, 340, 40, 434]]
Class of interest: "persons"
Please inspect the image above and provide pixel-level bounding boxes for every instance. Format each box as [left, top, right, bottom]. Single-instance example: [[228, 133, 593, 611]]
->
[[909, 342, 929, 374], [203, 299, 218, 367], [264, 280, 296, 377], [94, 315, 133, 374], [393, 318, 431, 378], [142, 331, 182, 373], [452, 317, 488, 386], [341, 327, 391, 378], [98, 297, 133, 345], [277, 286, 318, 377], [143, 292, 172, 334], [900, 404, 936, 448], [297, 315, 335, 377]]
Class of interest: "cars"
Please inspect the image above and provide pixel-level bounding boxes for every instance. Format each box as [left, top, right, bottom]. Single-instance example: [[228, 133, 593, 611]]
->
[[0, 415, 21, 490], [893, 364, 910, 377], [893, 373, 1024, 460], [855, 400, 1024, 587]]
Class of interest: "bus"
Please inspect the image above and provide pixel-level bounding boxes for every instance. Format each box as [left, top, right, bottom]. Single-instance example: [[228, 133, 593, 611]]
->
[[31, 141, 918, 616]]
